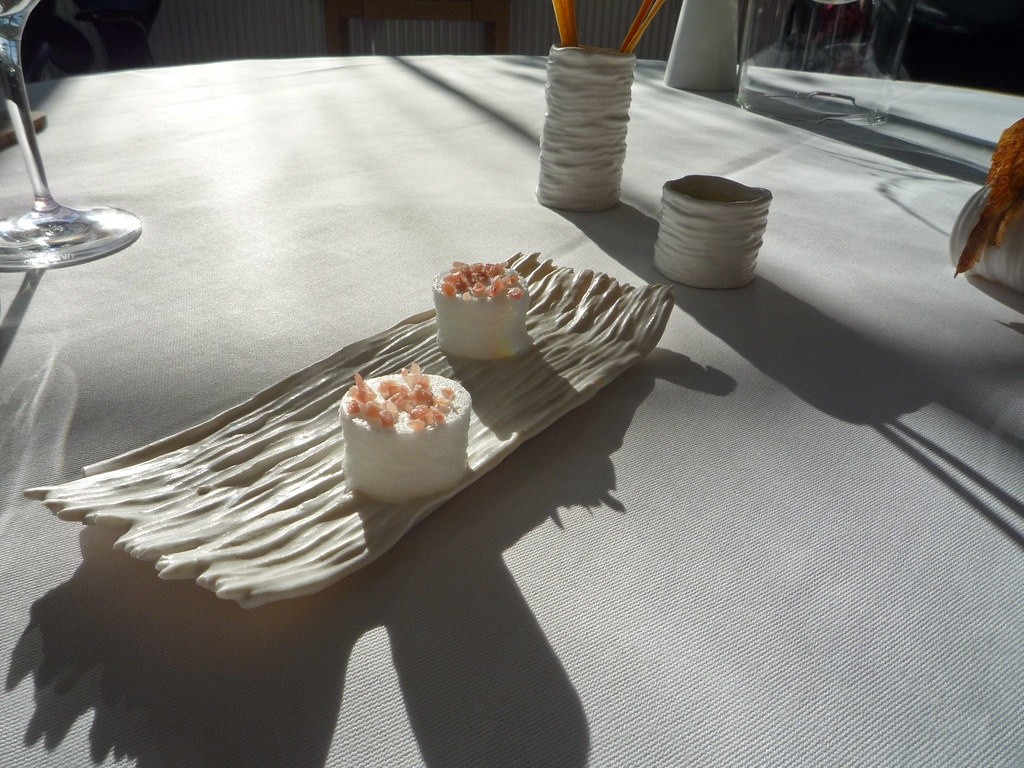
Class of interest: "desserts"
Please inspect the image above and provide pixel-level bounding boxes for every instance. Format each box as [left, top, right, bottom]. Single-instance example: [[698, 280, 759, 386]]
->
[[336, 363, 473, 504], [432, 260, 530, 360]]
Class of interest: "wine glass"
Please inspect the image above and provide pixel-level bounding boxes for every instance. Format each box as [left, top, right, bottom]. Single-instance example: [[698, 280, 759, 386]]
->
[[0, 0, 142, 271]]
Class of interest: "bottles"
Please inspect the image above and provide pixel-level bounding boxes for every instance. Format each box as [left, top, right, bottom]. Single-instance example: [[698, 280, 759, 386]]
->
[[663, 0, 739, 93]]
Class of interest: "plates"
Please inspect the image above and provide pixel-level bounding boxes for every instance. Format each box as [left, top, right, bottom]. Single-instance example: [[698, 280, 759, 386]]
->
[[22, 251, 675, 611]]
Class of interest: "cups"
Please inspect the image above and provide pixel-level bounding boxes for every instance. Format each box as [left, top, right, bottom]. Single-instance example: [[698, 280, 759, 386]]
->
[[948, 184, 1024, 300], [535, 42, 637, 212], [653, 174, 773, 290]]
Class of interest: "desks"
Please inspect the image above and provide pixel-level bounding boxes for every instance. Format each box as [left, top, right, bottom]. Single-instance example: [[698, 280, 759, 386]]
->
[[1, 54, 1024, 767]]
